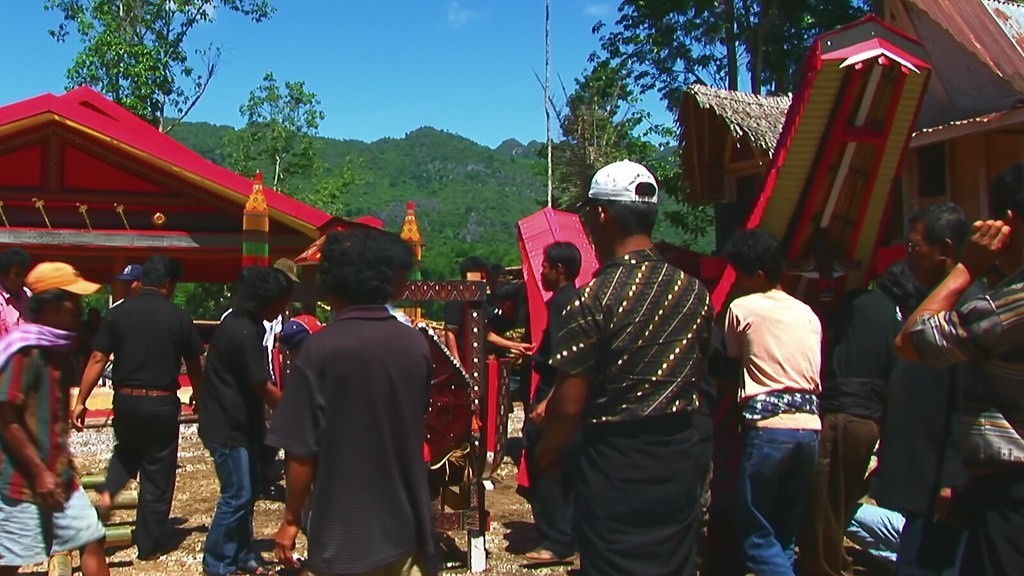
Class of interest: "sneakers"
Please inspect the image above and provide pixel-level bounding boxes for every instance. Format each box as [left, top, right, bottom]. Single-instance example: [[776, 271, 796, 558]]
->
[[433, 531, 467, 568]]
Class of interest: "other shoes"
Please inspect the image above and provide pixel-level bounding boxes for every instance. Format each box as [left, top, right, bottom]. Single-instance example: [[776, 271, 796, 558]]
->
[[94, 502, 115, 528]]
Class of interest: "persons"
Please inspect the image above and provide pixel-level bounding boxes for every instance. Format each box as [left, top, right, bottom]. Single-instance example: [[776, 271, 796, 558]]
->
[[274, 230, 440, 576], [0, 165, 1024, 576], [543, 161, 713, 575]]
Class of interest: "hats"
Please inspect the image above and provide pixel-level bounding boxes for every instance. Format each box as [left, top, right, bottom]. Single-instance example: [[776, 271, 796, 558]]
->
[[230, 266, 288, 314], [115, 264, 143, 281], [26, 260, 102, 295], [274, 258, 301, 283], [576, 159, 659, 203]]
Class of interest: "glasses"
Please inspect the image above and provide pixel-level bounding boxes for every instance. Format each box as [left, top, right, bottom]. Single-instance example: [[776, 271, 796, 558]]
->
[[907, 240, 938, 253]]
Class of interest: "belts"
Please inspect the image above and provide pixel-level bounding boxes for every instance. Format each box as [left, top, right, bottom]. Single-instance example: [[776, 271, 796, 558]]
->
[[113, 384, 177, 396]]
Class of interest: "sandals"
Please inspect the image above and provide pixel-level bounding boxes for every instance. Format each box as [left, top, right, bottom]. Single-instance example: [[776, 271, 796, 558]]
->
[[240, 556, 277, 576], [521, 545, 578, 562]]
